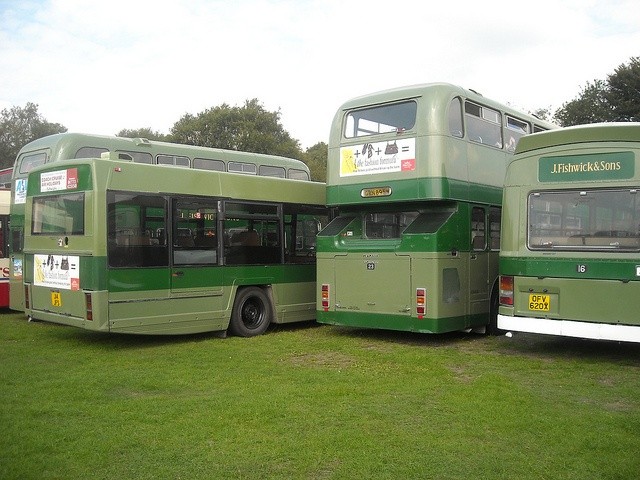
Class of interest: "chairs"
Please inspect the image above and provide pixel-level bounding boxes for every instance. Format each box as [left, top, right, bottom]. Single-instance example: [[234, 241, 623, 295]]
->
[[470, 136, 483, 143], [451, 130, 462, 138]]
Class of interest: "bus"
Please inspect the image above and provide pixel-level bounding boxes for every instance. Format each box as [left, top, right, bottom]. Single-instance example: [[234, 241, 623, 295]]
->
[[9, 133, 311, 311], [497, 122, 640, 342], [316, 84, 623, 333], [24, 158, 418, 338]]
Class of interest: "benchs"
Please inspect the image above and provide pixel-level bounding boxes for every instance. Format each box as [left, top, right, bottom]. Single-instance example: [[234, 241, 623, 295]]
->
[[115, 235, 150, 245], [175, 236, 194, 246], [584, 237, 640, 247], [196, 235, 231, 246], [239, 231, 261, 245], [530, 237, 583, 246]]
